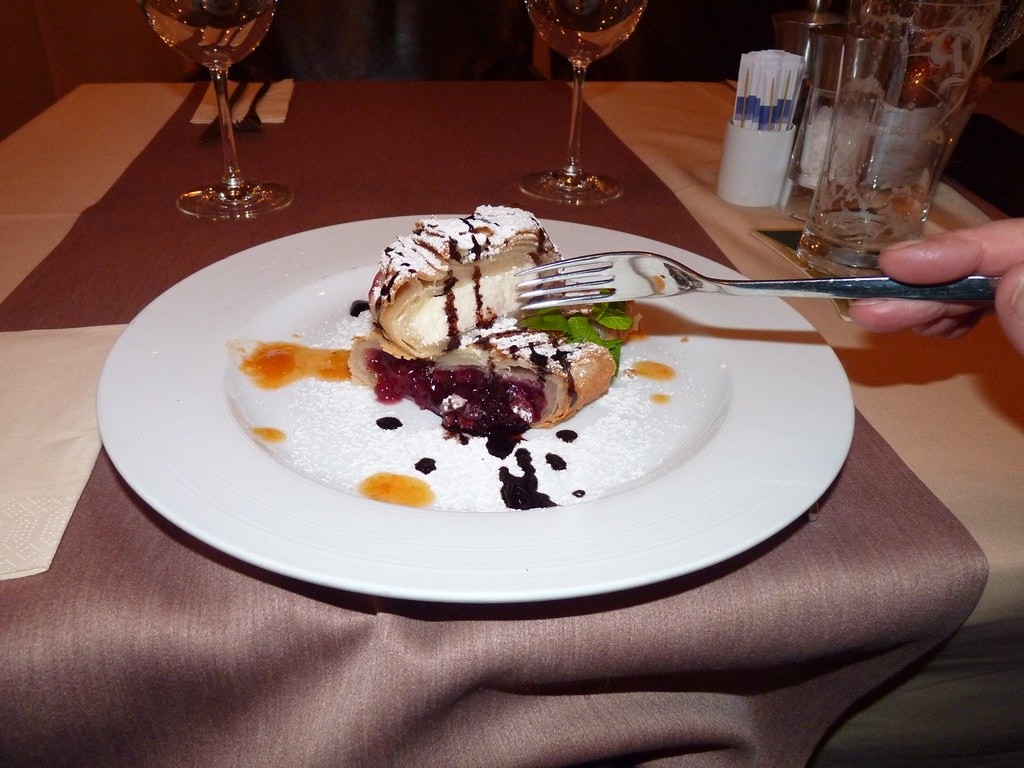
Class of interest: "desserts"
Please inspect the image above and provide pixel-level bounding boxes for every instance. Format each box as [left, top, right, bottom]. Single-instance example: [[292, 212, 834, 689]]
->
[[347, 316, 617, 435], [367, 205, 565, 356]]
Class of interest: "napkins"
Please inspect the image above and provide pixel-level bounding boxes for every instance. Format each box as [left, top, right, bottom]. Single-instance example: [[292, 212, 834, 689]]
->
[[1, 324, 128, 581], [191, 79, 295, 123]]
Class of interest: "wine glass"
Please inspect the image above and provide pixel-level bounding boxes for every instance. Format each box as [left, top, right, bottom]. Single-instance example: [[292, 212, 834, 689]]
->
[[518, 0, 647, 206], [143, 1, 293, 217]]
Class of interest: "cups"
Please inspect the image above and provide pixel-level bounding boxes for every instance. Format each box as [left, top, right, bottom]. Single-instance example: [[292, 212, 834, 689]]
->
[[795, 1, 998, 276], [715, 120, 796, 208], [778, 25, 845, 221], [773, 0, 844, 53]]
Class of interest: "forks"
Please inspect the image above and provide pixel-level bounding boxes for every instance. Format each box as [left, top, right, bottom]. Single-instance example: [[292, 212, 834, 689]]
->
[[231, 80, 273, 131], [515, 250, 1002, 312]]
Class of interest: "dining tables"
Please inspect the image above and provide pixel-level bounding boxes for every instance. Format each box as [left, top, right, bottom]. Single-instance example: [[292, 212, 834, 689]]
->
[[0, 83, 1023, 768]]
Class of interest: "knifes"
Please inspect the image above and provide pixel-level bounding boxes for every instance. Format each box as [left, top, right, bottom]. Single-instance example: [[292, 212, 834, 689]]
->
[[197, 79, 249, 144]]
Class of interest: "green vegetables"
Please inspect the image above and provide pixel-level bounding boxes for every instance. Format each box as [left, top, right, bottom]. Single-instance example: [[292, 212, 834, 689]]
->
[[512, 284, 633, 378]]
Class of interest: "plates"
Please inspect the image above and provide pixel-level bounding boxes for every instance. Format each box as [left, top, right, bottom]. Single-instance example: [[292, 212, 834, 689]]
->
[[97, 214, 856, 602]]
